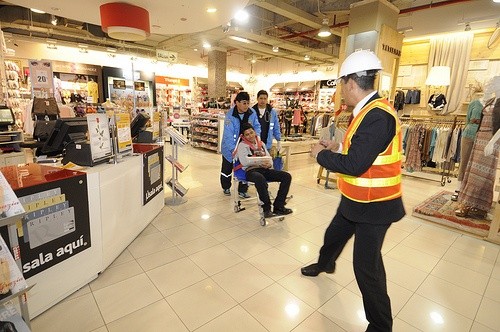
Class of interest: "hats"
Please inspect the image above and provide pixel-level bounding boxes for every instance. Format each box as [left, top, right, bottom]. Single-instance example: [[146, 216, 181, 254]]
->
[[234, 92, 250, 104]]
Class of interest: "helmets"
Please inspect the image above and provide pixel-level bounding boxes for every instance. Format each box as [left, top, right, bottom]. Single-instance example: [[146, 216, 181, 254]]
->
[[336, 49, 384, 79]]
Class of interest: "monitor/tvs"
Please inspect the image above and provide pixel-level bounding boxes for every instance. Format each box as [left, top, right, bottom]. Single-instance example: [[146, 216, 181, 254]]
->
[[0, 107, 15, 132], [130, 111, 149, 138], [41, 117, 88, 158]]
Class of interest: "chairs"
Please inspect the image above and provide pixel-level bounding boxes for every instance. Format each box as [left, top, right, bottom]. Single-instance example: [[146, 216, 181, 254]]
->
[[233, 154, 294, 226]]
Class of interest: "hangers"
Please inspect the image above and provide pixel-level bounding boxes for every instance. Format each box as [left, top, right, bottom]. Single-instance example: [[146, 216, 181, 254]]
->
[[400, 118, 463, 134], [313, 111, 348, 121]]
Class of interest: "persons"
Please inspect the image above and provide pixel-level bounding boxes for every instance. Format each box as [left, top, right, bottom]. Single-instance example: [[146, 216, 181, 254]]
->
[[220, 92, 261, 199], [452, 76, 500, 218], [238, 123, 294, 218], [252, 90, 282, 155], [301, 50, 406, 332], [284, 106, 301, 137]]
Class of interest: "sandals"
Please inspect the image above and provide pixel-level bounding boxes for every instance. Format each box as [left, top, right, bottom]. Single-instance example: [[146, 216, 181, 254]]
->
[[451, 190, 460, 200]]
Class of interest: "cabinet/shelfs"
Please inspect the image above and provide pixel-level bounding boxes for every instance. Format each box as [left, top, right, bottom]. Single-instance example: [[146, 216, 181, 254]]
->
[[270, 93, 312, 134], [155, 86, 232, 206], [0, 29, 37, 138], [318, 89, 338, 109]]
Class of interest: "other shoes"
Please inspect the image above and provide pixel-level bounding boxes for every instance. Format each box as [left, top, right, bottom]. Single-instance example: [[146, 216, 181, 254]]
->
[[238, 192, 251, 198], [273, 207, 292, 215], [224, 189, 231, 196], [264, 209, 271, 217], [455, 209, 466, 216]]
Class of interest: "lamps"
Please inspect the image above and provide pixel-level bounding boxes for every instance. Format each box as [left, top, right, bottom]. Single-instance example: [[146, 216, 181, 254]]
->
[[304, 54, 311, 60], [272, 44, 279, 52], [317, 17, 331, 37], [426, 66, 450, 90], [250, 54, 256, 62]]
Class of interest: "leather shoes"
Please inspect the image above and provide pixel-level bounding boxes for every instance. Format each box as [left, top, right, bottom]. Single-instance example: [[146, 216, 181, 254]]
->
[[301, 263, 336, 276]]
